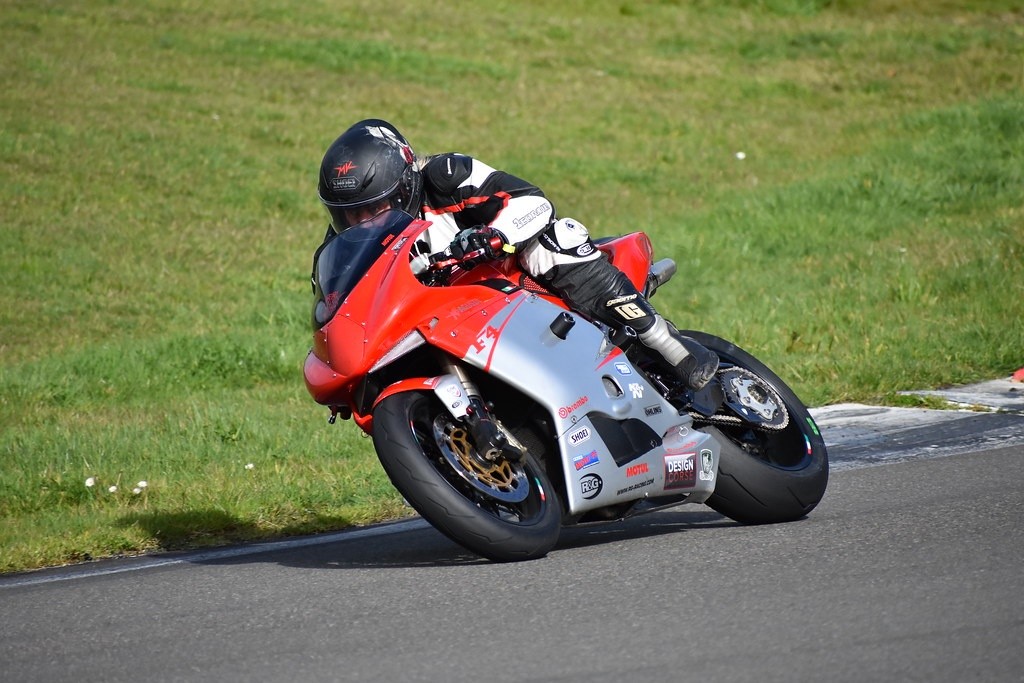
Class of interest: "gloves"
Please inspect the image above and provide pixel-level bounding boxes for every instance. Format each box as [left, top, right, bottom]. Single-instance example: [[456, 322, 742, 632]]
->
[[451, 224, 505, 273]]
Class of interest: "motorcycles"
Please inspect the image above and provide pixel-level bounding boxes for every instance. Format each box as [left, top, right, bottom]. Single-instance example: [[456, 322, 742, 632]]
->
[[303, 207, 829, 563]]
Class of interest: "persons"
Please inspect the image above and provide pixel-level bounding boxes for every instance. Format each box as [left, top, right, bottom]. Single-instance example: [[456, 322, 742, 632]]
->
[[309, 118, 722, 391]]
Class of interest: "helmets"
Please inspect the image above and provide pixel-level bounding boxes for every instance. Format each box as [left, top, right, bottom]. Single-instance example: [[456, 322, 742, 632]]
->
[[317, 118, 421, 245]]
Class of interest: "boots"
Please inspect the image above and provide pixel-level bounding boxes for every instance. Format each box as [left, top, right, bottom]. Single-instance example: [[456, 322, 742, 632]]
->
[[592, 274, 719, 390]]
